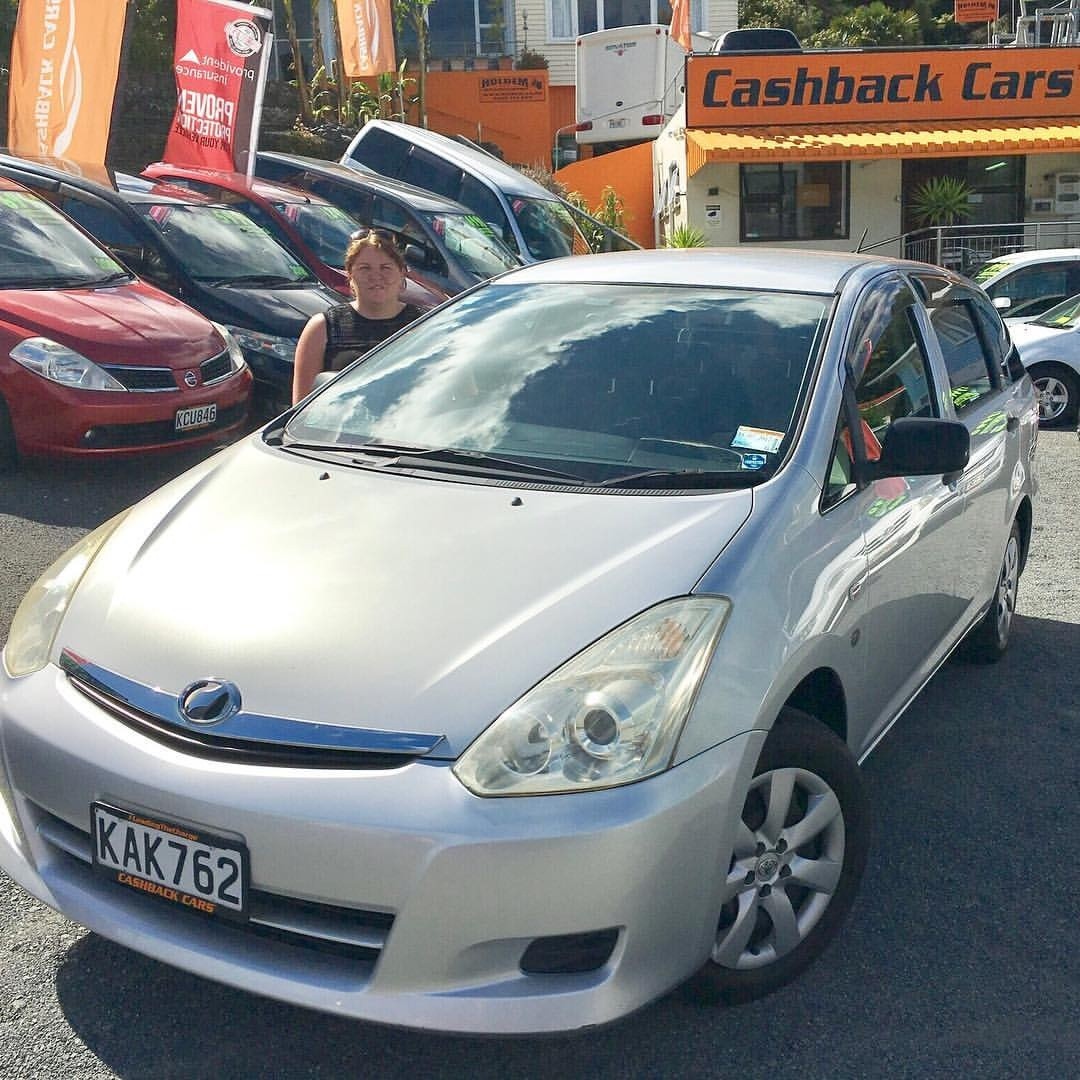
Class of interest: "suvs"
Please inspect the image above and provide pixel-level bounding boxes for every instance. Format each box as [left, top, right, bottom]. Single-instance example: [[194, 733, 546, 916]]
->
[[256, 151, 520, 294], [969, 249, 1080, 317]]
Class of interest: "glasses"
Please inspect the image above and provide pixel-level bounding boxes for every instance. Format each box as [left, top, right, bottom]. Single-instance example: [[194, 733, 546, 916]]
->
[[350, 227, 392, 242]]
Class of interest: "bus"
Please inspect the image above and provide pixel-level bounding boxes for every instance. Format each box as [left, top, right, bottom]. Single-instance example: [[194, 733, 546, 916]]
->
[[574, 24, 714, 145]]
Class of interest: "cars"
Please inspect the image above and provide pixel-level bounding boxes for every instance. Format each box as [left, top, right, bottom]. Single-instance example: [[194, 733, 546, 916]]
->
[[0, 248, 1044, 1040], [138, 162, 453, 311], [0, 178, 254, 472], [1001, 295, 1080, 425], [0, 154, 355, 394]]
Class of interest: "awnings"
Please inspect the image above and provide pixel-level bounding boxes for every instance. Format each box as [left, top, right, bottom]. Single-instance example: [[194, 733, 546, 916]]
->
[[686, 116, 1080, 177]]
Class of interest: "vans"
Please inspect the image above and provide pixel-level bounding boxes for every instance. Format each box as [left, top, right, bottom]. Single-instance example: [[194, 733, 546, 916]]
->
[[336, 118, 596, 266], [708, 27, 805, 54]]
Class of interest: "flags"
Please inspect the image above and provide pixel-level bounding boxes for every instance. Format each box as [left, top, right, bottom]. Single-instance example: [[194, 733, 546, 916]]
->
[[336, 0, 397, 78], [8, 0, 127, 164], [669, 0, 689, 51], [160, 0, 275, 173]]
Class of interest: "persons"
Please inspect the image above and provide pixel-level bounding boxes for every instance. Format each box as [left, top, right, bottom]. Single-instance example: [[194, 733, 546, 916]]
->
[[293, 229, 421, 407]]
